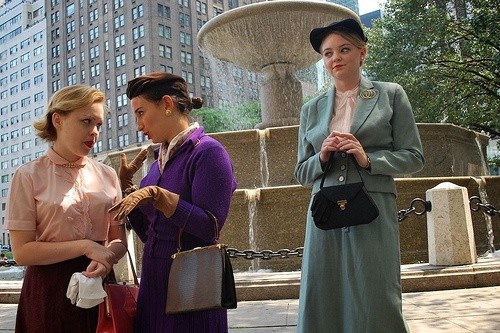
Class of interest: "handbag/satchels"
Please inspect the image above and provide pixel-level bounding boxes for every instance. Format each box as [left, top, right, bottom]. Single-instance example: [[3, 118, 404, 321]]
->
[[310, 151, 379, 230], [163, 209, 238, 314], [95, 241, 140, 333]]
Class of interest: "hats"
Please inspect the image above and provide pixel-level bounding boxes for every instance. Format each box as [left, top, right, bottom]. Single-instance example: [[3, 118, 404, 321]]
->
[[309, 19, 369, 53]]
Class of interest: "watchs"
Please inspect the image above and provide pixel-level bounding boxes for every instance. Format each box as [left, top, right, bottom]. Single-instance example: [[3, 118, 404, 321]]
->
[[366, 157, 371, 171]]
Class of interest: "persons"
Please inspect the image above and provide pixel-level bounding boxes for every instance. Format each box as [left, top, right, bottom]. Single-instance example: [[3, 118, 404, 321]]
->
[[3, 84, 127, 333], [106, 72, 238, 333], [293, 18, 426, 333]]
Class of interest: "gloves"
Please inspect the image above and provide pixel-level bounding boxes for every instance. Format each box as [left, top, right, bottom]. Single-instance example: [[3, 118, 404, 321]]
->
[[65, 270, 106, 309], [107, 185, 180, 224], [117, 149, 149, 197]]
